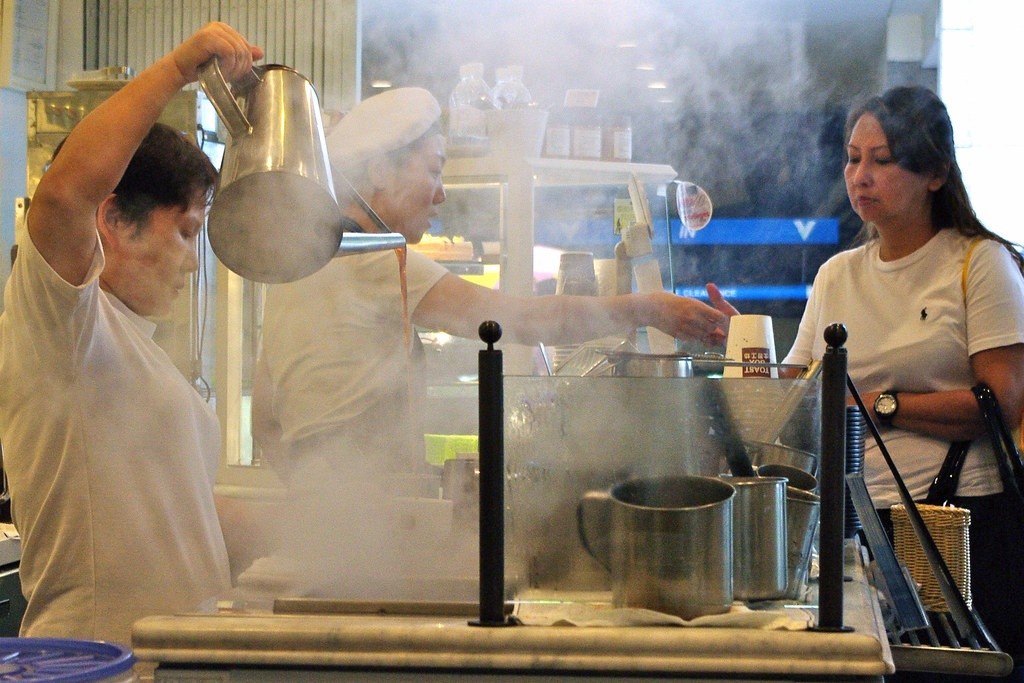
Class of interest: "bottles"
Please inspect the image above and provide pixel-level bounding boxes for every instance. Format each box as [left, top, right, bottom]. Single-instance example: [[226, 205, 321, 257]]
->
[[447, 63, 532, 150]]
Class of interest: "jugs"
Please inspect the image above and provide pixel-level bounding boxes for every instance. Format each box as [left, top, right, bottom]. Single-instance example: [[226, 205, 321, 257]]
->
[[198, 54, 407, 283]]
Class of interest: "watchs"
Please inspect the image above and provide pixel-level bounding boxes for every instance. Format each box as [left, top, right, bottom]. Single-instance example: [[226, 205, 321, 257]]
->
[[872, 388, 899, 427]]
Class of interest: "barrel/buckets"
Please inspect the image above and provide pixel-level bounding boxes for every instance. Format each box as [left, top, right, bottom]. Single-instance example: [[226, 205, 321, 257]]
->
[[0, 637, 134, 683], [594, 349, 693, 377]]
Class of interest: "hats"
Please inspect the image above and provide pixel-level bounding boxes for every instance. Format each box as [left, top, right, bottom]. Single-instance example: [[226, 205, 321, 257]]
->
[[326, 87, 443, 158]]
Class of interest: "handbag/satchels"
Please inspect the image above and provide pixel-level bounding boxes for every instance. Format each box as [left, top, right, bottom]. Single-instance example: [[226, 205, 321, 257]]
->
[[924, 383, 1024, 627]]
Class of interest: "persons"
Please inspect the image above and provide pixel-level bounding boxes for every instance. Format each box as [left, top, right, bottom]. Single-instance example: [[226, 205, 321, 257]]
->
[[706, 86, 1024, 683], [252, 87, 729, 540], [0, 20, 263, 652]]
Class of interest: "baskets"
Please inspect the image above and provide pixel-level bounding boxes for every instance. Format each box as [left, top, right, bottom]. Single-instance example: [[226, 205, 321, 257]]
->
[[891, 502, 972, 612]]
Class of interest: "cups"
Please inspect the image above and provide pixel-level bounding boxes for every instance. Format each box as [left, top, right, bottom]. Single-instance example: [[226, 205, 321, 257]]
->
[[721, 314, 780, 379], [552, 253, 601, 377], [576, 437, 820, 621], [375, 459, 514, 602]]
